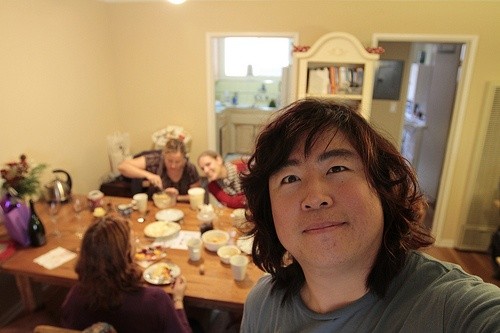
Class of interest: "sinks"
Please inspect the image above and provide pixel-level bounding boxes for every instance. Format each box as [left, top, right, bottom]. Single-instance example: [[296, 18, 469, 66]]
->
[[228, 99, 279, 126]]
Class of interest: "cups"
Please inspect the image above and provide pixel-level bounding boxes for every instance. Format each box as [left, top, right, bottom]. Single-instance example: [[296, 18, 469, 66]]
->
[[87, 190, 104, 213], [230, 255, 248, 281], [131, 193, 148, 210], [186, 238, 202, 261], [188, 187, 206, 210], [197, 212, 214, 234]]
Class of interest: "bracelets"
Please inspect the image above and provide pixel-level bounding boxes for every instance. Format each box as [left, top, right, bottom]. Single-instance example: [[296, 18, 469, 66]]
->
[[173, 298, 183, 302]]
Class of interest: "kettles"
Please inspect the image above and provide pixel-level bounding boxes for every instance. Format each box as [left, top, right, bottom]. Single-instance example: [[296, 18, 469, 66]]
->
[[44, 169, 72, 204]]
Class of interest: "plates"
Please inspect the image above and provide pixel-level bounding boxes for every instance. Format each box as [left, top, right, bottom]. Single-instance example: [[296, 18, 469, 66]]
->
[[142, 262, 181, 284], [144, 221, 180, 239], [236, 234, 260, 254], [155, 208, 184, 222]]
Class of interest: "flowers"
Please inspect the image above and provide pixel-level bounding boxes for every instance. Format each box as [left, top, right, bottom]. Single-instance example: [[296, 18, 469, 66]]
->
[[0, 154, 48, 195]]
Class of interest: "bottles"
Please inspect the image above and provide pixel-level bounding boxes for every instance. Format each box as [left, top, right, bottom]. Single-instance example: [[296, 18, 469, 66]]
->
[[28, 199, 47, 248]]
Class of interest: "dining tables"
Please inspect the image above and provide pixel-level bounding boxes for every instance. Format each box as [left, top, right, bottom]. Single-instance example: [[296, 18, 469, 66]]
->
[[0, 192, 275, 316]]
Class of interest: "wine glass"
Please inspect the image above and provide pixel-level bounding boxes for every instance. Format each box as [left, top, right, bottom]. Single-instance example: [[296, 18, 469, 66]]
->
[[70, 194, 86, 233], [49, 199, 62, 237]]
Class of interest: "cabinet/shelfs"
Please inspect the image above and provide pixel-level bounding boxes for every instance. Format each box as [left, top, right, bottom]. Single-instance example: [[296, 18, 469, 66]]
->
[[293, 31, 385, 123], [216, 122, 264, 158]]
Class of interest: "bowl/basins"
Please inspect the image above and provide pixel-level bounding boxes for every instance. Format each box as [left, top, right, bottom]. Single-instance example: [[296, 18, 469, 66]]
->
[[152, 191, 177, 209], [217, 246, 241, 264], [202, 230, 230, 251]]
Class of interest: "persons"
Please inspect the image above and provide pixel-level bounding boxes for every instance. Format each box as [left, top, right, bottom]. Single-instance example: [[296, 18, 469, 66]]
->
[[241, 97, 500, 333], [197, 151, 256, 209], [50, 217, 193, 333], [118, 138, 200, 202]]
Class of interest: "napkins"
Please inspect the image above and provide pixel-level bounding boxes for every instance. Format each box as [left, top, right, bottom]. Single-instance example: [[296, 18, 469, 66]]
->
[[34, 246, 76, 270]]
